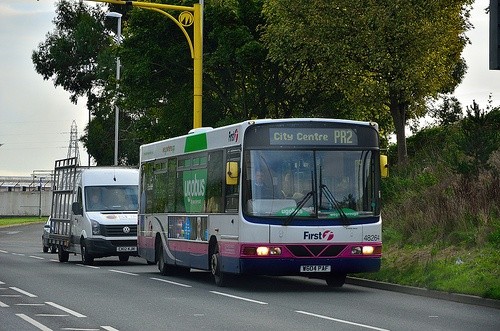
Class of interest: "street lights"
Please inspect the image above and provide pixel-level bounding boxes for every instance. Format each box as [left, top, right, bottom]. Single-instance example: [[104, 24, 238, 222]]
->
[[105, 12, 123, 167]]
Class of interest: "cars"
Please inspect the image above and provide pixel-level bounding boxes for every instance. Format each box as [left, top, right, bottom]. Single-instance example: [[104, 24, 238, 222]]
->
[[42, 212, 71, 254]]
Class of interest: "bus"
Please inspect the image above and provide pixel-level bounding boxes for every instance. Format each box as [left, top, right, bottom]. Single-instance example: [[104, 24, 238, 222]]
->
[[135, 117, 393, 288]]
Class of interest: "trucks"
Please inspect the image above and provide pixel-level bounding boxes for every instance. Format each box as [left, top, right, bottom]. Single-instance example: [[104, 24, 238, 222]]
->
[[47, 157, 159, 266]]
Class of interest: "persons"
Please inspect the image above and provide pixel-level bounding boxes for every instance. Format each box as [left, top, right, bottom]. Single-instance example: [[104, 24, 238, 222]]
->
[[251, 170, 271, 200]]
